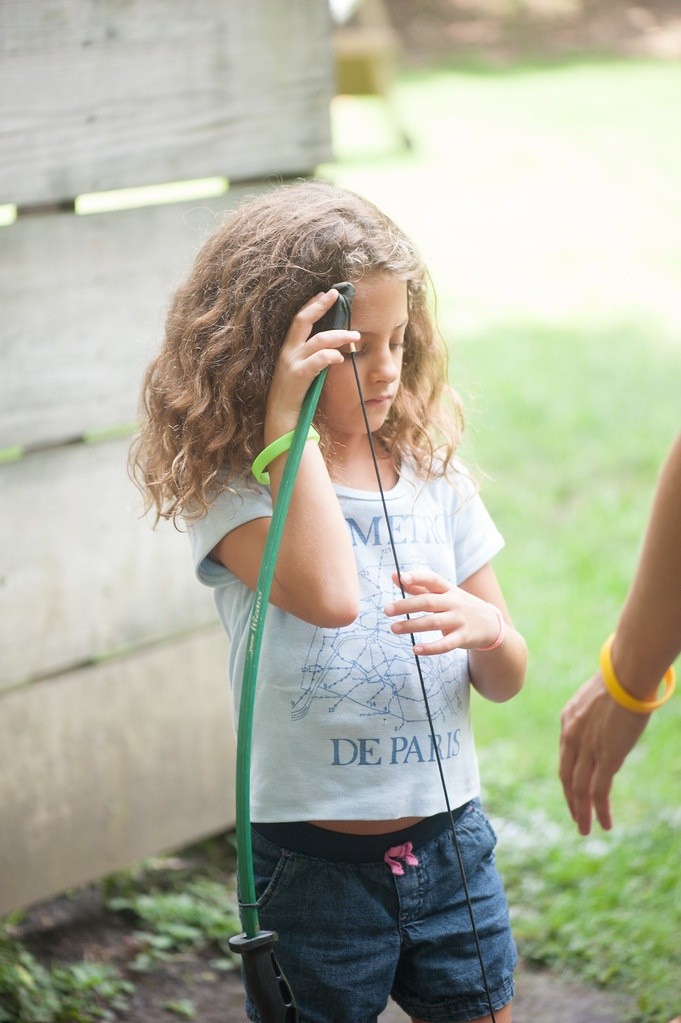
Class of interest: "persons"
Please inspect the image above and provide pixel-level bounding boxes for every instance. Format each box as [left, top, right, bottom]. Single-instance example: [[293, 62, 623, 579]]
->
[[126, 181, 529, 1021], [558, 430, 681, 836]]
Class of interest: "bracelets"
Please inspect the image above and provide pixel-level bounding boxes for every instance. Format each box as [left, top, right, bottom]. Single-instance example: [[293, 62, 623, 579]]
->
[[251, 425, 321, 485], [600, 630, 675, 713], [472, 603, 505, 652]]
